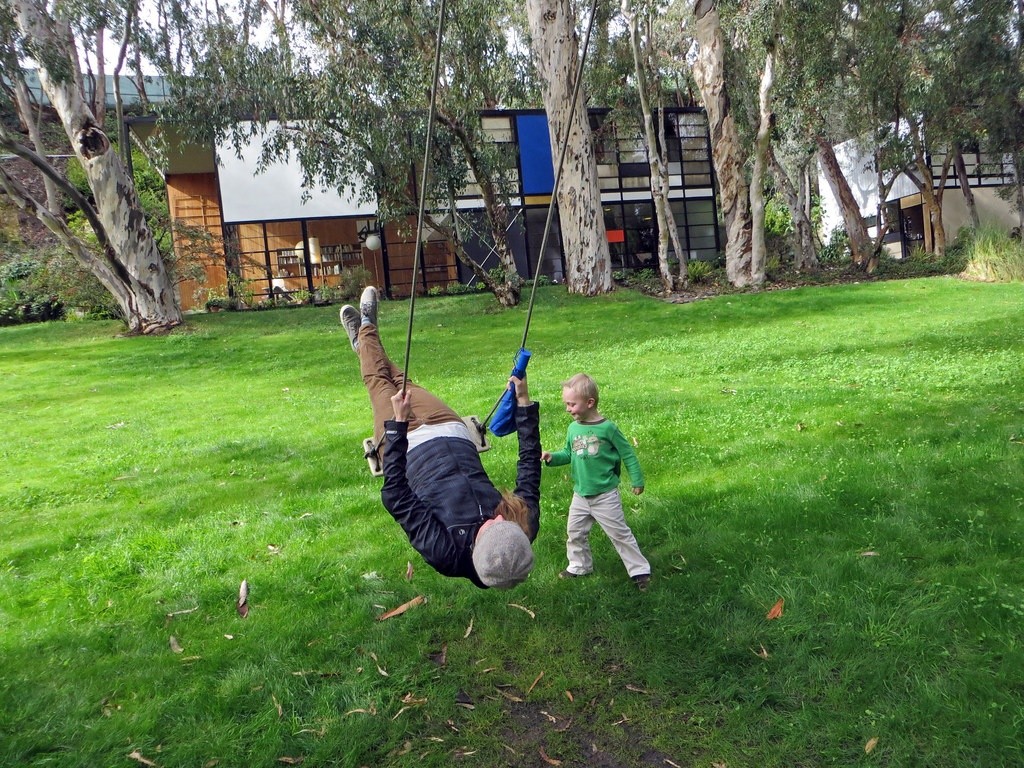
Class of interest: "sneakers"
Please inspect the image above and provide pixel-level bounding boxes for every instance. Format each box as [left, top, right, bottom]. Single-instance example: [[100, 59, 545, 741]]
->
[[360, 286, 379, 325], [340, 305, 361, 352]]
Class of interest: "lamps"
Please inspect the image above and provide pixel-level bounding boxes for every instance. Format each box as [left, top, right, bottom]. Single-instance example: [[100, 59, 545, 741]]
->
[[366, 234, 381, 251], [308, 236, 321, 265], [295, 241, 304, 259]]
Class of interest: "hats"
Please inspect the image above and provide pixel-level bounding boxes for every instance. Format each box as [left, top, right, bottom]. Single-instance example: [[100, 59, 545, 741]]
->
[[472, 521, 534, 589]]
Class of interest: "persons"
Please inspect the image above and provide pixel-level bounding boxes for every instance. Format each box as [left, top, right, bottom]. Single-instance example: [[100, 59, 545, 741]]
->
[[539, 371, 652, 588], [339, 284, 543, 591]]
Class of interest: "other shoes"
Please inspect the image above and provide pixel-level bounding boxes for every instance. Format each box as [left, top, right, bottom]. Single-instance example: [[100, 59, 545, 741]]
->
[[636, 574, 650, 591], [558, 570, 592, 580]]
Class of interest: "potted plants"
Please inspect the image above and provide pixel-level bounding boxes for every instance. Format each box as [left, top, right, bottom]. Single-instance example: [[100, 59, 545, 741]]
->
[[290, 286, 364, 305], [203, 296, 236, 313], [389, 286, 401, 299]]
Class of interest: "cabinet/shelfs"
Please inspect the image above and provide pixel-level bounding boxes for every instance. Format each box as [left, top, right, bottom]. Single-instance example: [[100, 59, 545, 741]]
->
[[277, 244, 365, 289]]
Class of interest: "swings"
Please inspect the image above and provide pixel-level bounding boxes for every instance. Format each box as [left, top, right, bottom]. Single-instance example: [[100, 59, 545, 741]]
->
[[361, 1, 600, 480]]
